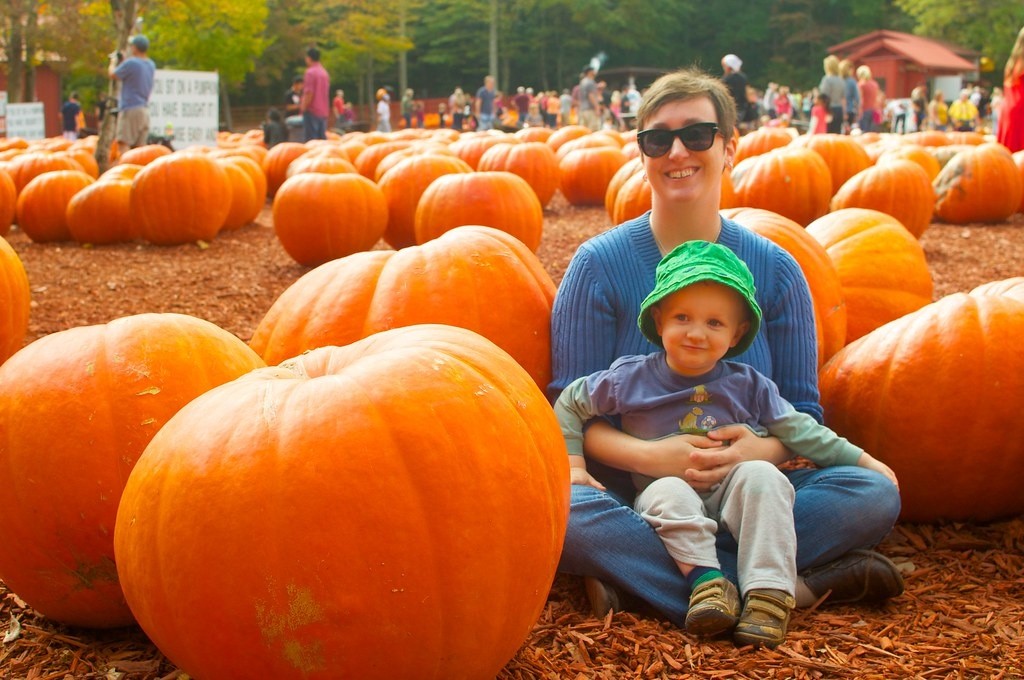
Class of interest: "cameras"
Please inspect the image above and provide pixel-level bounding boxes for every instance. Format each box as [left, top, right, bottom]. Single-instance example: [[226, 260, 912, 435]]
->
[[108, 51, 122, 60]]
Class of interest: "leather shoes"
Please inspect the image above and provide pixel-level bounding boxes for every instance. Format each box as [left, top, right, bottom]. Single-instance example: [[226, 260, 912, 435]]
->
[[583, 579, 634, 619], [802, 548, 905, 607]]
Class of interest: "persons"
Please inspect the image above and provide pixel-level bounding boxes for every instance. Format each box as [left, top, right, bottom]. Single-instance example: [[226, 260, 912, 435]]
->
[[545, 62, 906, 619], [578, 65, 601, 133], [718, 54, 749, 128], [400, 87, 425, 129], [552, 238, 900, 646], [107, 33, 156, 156], [375, 88, 392, 133], [996, 26, 1024, 154], [474, 75, 503, 132], [262, 46, 331, 151], [332, 89, 355, 135], [438, 54, 1004, 137], [56, 90, 106, 142]]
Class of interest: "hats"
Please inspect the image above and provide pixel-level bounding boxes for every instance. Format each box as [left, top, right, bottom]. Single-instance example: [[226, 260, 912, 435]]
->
[[127, 34, 149, 52], [583, 65, 594, 72], [636, 240, 763, 360]]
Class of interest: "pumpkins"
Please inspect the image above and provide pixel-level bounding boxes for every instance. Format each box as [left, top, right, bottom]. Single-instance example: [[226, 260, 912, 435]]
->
[[0, 108, 1024, 680]]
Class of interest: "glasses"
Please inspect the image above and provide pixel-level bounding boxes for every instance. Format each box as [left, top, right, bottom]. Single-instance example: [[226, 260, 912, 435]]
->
[[637, 122, 728, 157]]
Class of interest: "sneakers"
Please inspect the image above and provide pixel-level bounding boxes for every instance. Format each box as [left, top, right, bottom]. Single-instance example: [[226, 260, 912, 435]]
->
[[732, 587, 796, 648], [684, 576, 741, 637]]
